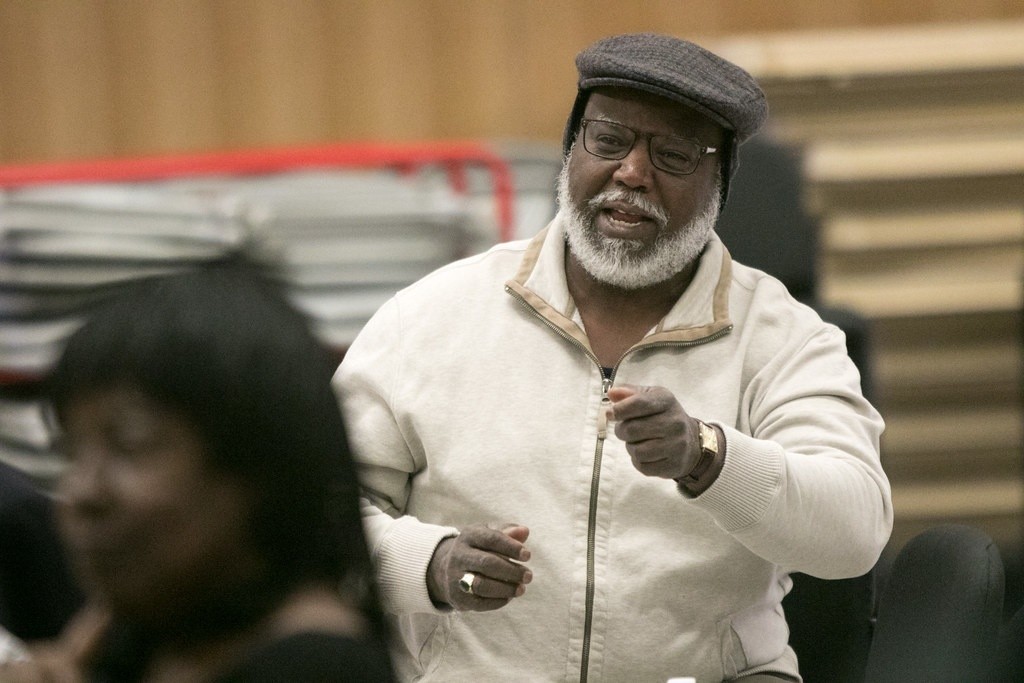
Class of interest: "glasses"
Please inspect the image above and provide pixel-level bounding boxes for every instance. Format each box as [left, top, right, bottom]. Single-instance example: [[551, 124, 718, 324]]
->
[[579, 116, 722, 176]]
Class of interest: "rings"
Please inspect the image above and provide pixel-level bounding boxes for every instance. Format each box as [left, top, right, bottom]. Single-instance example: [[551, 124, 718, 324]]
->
[[459, 573, 475, 594]]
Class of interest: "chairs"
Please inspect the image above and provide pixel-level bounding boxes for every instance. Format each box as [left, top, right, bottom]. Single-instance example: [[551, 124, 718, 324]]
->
[[863, 524, 1005, 683]]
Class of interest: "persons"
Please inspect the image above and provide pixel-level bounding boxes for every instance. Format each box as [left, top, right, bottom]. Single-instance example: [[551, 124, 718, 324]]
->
[[330, 31, 897, 682], [0, 251, 396, 682]]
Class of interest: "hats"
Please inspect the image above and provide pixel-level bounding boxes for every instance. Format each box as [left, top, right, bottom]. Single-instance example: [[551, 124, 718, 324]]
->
[[576, 34, 768, 148]]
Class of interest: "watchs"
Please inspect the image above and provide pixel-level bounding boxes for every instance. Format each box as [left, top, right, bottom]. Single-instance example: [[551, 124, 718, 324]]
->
[[672, 417, 722, 483]]
